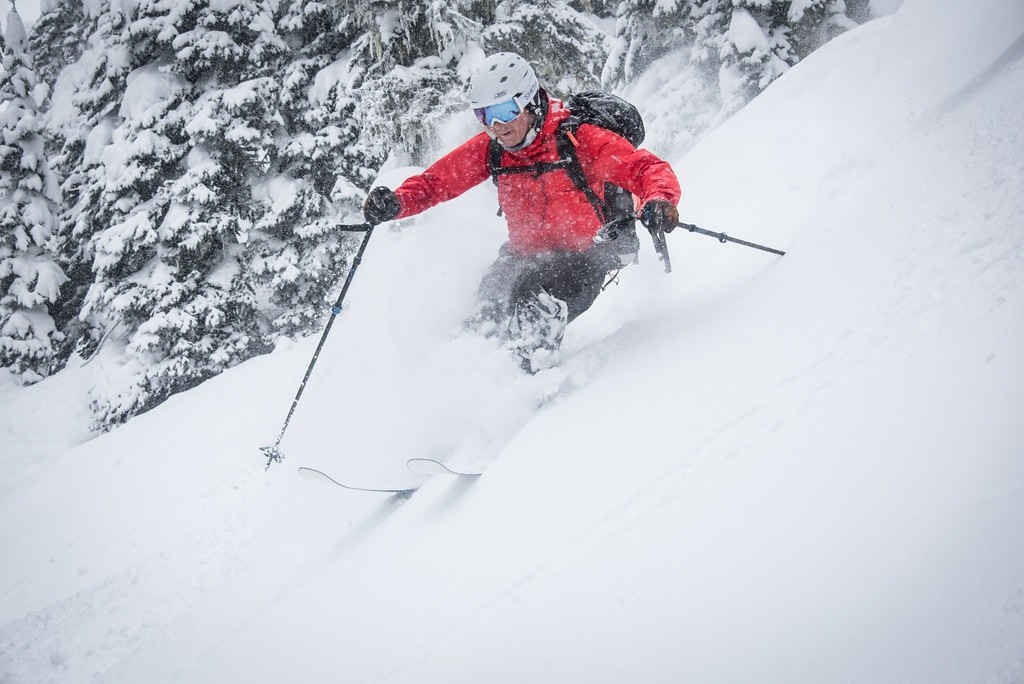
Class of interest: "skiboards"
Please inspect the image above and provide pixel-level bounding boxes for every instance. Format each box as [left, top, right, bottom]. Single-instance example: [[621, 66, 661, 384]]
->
[[293, 456, 484, 494]]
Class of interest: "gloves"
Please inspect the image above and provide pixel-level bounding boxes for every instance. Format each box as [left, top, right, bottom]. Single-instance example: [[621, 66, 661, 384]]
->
[[644, 200, 680, 233], [364, 186, 401, 225]]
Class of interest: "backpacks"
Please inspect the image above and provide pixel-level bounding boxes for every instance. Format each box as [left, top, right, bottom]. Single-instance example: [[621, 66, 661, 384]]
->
[[486, 90, 646, 255]]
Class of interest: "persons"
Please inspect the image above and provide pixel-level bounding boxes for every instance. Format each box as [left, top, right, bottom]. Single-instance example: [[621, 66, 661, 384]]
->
[[363, 51, 682, 377]]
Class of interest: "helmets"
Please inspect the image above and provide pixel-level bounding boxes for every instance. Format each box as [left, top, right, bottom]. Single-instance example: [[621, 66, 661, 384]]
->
[[470, 51, 539, 111]]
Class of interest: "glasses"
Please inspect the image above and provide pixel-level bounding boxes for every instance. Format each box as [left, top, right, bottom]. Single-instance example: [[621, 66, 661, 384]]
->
[[474, 97, 522, 127]]
